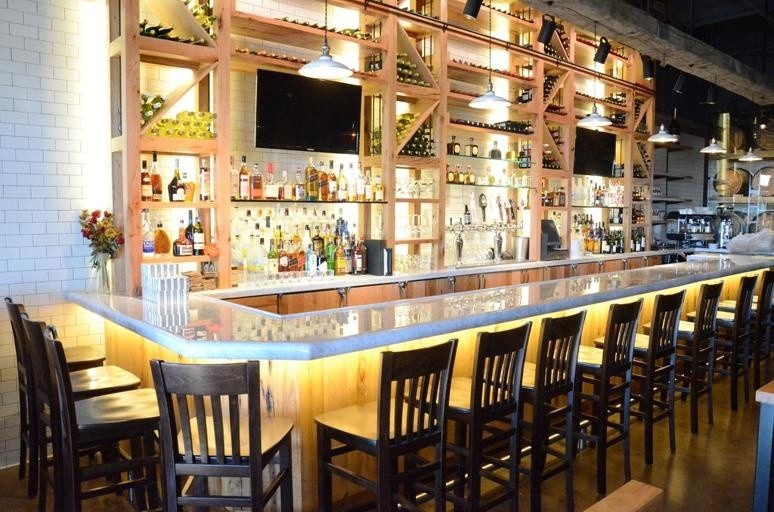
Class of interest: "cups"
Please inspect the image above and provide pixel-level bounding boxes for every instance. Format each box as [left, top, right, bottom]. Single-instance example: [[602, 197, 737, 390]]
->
[[510, 236, 528, 261], [651, 208, 659, 217]]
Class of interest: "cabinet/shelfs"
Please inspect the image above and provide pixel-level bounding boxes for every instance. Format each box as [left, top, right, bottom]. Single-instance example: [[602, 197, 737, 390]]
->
[[108, 1, 665, 318], [650, 140, 695, 247]]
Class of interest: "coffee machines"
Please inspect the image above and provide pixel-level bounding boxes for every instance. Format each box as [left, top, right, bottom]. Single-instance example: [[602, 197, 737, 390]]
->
[[665, 208, 715, 241]]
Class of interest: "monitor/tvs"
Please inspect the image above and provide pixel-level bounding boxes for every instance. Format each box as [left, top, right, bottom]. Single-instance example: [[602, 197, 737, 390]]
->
[[255, 68, 362, 155], [541, 219, 568, 251], [573, 127, 616, 177]]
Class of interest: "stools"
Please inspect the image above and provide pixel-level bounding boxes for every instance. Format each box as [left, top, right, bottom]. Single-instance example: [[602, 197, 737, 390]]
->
[[471, 308, 589, 512], [147, 356, 299, 512], [545, 296, 645, 496], [593, 288, 688, 467], [3, 295, 106, 496], [404, 320, 533, 512], [43, 322, 165, 512], [684, 267, 773, 414], [637, 279, 726, 437], [19, 312, 142, 512], [311, 336, 460, 512]]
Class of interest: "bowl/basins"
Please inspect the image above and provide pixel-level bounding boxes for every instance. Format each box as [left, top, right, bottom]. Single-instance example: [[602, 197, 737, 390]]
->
[[708, 244, 718, 250]]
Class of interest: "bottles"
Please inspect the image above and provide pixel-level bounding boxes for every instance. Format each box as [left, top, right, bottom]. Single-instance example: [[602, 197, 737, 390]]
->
[[572, 176, 609, 207], [234, 47, 308, 65], [448, 135, 460, 154], [634, 100, 640, 119], [455, 234, 462, 258], [631, 162, 647, 252], [569, 277, 600, 292], [542, 143, 562, 169], [231, 205, 367, 288], [395, 111, 436, 157], [138, 19, 206, 45], [281, 17, 337, 32], [371, 53, 432, 90], [543, 73, 567, 116], [612, 113, 624, 126], [229, 151, 385, 201], [144, 264, 188, 294], [450, 119, 533, 135], [230, 309, 351, 341], [482, 3, 535, 23], [337, 28, 359, 36], [574, 214, 624, 253], [550, 127, 563, 146], [185, 2, 215, 41], [139, 151, 209, 201], [487, 79, 492, 92], [541, 183, 565, 207], [636, 127, 649, 134], [543, 31, 570, 59], [465, 137, 476, 156], [141, 208, 204, 257], [447, 164, 527, 187], [354, 33, 372, 40], [493, 230, 502, 258], [575, 91, 626, 106], [453, 58, 533, 82], [401, 7, 438, 20], [489, 141, 501, 159], [637, 141, 651, 170], [523, 43, 533, 49], [141, 299, 189, 334], [142, 94, 217, 142], [464, 204, 470, 224]]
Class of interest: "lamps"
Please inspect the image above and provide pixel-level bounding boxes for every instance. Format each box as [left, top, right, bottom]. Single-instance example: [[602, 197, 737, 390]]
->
[[698, 74, 728, 153], [647, 59, 680, 145], [738, 94, 763, 162]]
